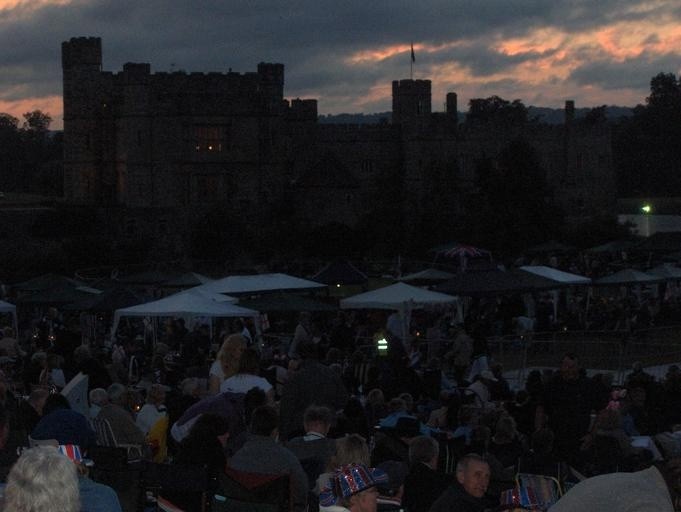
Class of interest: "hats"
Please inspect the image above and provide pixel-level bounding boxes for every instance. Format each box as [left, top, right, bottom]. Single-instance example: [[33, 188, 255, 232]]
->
[[57, 444, 94, 467], [501, 473, 575, 512], [319, 463, 389, 507]]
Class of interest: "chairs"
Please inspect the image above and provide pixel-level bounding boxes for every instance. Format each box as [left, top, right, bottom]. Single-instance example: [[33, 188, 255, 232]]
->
[[95, 417, 143, 463], [455, 387, 486, 425]]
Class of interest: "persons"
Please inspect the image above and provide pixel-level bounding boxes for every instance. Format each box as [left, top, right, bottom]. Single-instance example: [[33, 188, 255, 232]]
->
[[0, 245, 680, 511]]
